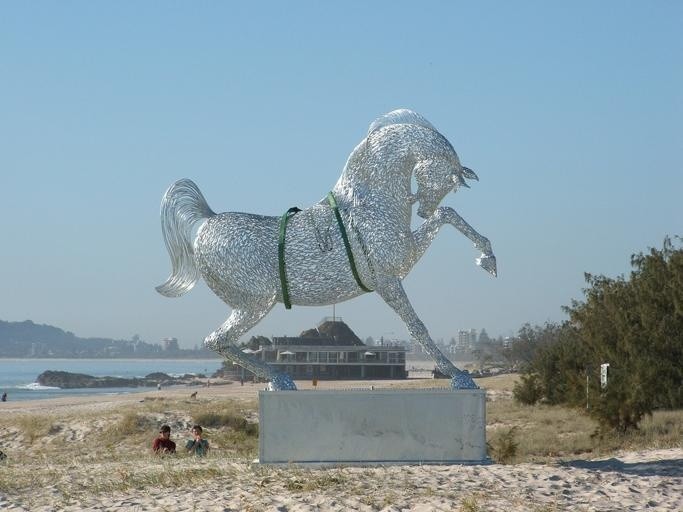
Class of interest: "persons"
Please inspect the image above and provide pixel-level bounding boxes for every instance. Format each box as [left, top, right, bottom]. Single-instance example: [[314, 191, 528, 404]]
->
[[1, 391, 7, 401], [186, 425, 209, 457], [152, 425, 176, 453], [157, 383, 161, 391], [190, 391, 197, 399]]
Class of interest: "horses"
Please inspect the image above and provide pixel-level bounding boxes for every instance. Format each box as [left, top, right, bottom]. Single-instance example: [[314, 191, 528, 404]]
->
[[155, 107, 499, 391]]
